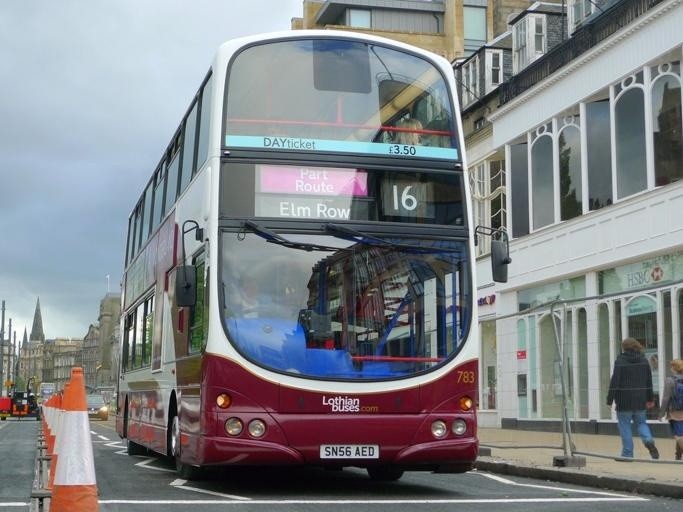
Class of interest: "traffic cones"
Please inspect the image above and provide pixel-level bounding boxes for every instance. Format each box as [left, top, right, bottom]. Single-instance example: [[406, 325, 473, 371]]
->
[[37, 367, 99, 512]]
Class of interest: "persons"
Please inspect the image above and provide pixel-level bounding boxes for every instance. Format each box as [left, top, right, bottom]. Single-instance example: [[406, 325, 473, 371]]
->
[[656, 359, 683, 460], [392, 119, 423, 144], [606, 338, 659, 461], [228, 277, 274, 319]]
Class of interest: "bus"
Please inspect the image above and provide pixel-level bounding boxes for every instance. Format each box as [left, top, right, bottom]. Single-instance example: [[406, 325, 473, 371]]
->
[[114, 27, 512, 487]]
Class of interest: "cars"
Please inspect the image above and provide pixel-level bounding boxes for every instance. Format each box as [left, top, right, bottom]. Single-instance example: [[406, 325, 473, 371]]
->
[[86, 394, 110, 421]]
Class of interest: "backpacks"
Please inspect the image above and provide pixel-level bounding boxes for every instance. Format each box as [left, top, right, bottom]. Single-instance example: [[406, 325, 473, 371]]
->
[[670, 376, 683, 410]]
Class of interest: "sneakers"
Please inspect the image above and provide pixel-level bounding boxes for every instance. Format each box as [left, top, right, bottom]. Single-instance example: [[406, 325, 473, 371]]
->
[[646, 442, 658, 458]]
